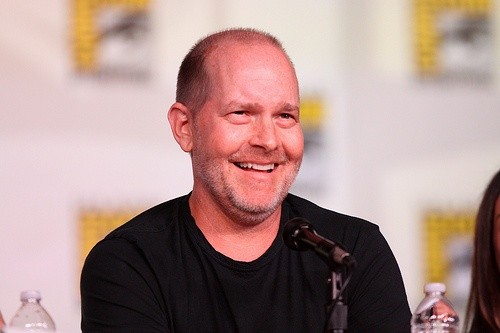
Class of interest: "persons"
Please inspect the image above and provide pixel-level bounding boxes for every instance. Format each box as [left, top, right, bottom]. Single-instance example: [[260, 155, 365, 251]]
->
[[462, 170, 500, 333], [78, 27, 413, 333]]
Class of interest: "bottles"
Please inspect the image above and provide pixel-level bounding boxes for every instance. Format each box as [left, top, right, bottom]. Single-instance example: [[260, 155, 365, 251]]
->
[[3, 290, 56, 333], [410, 283, 461, 333]]
